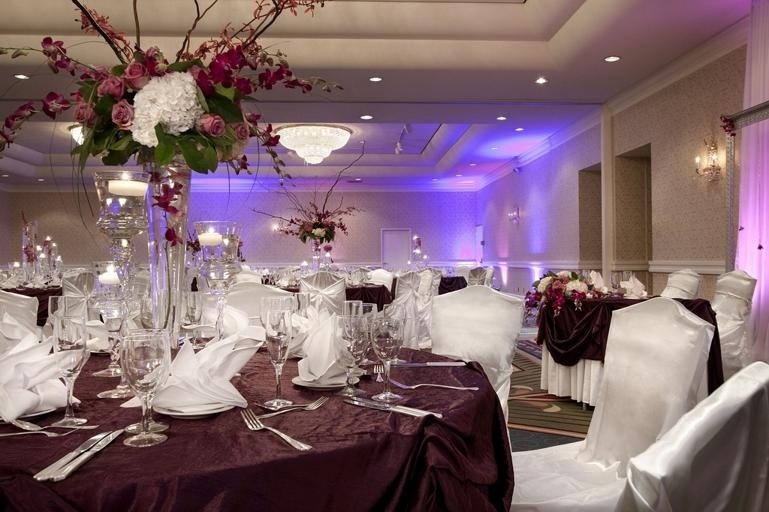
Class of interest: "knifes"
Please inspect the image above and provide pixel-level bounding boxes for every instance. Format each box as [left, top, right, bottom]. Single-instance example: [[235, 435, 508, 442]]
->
[[50, 429, 125, 482], [396, 361, 466, 368], [344, 399, 425, 418], [350, 397, 443, 420], [31, 431, 112, 482]]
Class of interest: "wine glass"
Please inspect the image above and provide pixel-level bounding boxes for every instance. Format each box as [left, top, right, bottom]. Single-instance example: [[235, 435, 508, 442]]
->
[[120, 337, 169, 449], [50, 318, 89, 427], [93, 290, 126, 377], [612, 271, 632, 297], [264, 310, 294, 410], [259, 297, 294, 367], [335, 299, 408, 403], [123, 330, 169, 435], [91, 169, 154, 368], [193, 219, 243, 353]]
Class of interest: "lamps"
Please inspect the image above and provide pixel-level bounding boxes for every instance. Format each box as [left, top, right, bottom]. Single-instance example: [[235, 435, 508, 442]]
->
[[690, 136, 723, 187]]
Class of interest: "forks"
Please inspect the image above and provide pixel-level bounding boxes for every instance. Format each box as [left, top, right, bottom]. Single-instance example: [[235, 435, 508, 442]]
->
[[239, 408, 312, 452], [254, 396, 329, 420], [388, 377, 480, 392]]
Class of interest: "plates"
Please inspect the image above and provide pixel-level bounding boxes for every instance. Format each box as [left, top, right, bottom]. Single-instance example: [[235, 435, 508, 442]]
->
[[291, 376, 360, 392], [152, 405, 236, 420], [0, 409, 57, 422]]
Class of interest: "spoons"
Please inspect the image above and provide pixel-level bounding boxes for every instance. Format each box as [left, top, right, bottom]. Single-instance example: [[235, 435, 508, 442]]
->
[[256, 399, 311, 412]]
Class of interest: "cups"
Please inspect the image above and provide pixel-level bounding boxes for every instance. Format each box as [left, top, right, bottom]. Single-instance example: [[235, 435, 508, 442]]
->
[[47, 295, 88, 337]]
